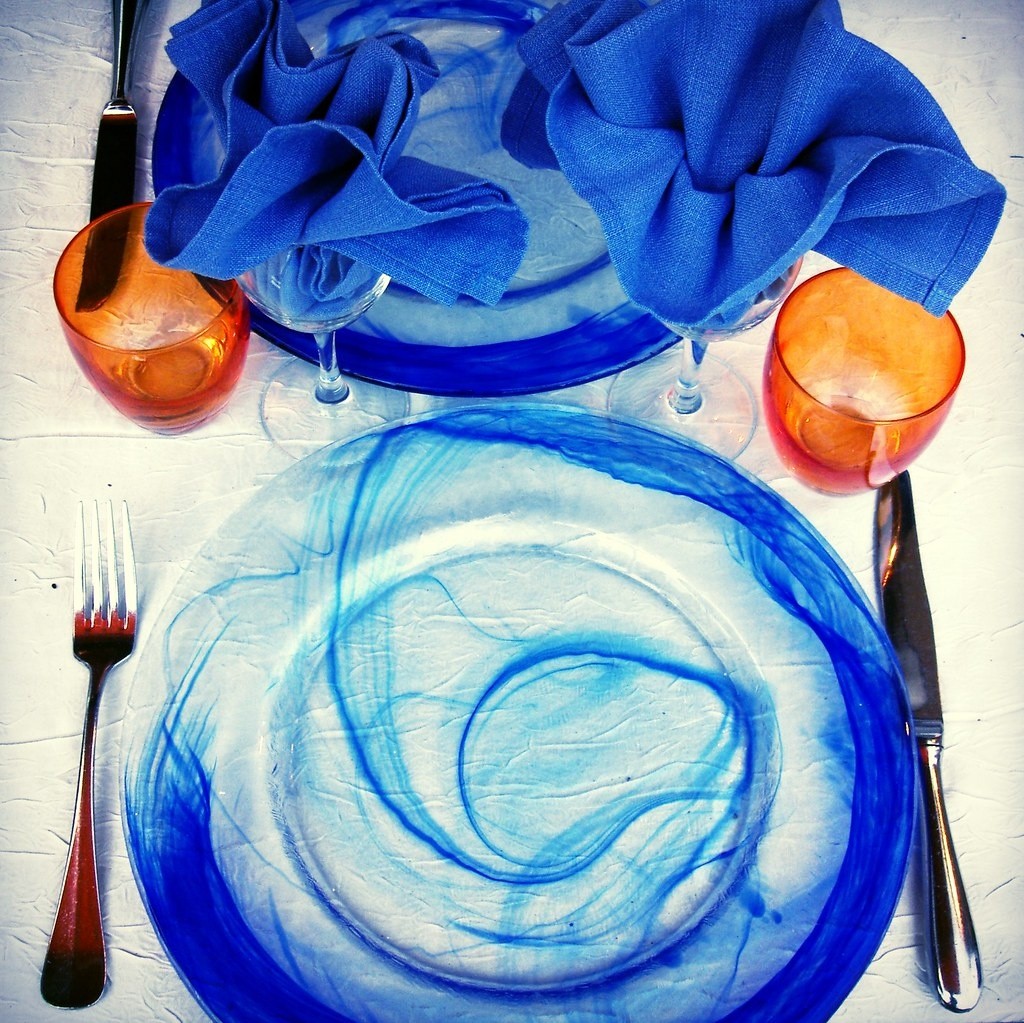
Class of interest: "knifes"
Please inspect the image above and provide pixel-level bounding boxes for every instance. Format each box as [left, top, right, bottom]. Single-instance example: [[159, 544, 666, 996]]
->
[[73, 0, 150, 314], [871, 472, 983, 1013]]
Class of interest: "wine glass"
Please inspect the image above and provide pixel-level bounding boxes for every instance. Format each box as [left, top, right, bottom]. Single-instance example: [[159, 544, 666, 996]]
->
[[234, 242, 413, 461], [607, 256, 806, 462]]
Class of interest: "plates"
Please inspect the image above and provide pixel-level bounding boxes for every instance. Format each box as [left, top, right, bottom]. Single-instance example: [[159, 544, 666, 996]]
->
[[120, 407, 921, 1023], [151, 0, 683, 397]]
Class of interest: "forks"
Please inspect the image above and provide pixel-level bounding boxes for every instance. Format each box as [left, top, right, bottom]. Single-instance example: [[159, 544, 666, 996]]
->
[[39, 500, 137, 1009]]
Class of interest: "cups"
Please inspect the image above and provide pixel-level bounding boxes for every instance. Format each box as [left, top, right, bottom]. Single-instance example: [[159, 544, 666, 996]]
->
[[51, 201, 251, 437], [761, 268, 967, 499]]
